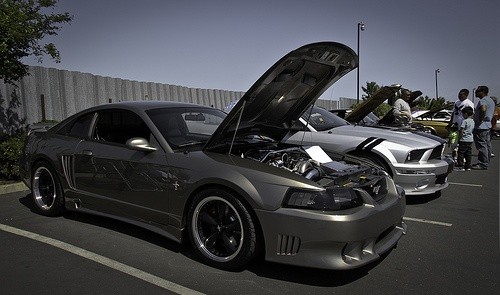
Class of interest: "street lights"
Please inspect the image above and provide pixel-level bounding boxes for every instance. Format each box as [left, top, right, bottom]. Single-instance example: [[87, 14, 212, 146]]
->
[[357, 20, 365, 103], [435, 68, 440, 99]]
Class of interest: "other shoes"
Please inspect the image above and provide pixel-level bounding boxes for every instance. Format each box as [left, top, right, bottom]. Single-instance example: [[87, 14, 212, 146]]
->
[[467, 167, 471, 170], [472, 165, 488, 170], [491, 154, 495, 156], [456, 166, 466, 171]]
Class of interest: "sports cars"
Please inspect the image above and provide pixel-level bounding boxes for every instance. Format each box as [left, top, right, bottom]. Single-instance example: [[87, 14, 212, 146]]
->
[[20, 40, 409, 270], [224, 95, 456, 195]]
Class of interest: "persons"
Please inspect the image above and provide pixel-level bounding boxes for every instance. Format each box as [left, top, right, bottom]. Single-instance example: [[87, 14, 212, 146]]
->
[[472, 86, 500, 170], [393, 89, 412, 126], [454, 107, 475, 171], [448, 123, 458, 160], [445, 88, 475, 165]]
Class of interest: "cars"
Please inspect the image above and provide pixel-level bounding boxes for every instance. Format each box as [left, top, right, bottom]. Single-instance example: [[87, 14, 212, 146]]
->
[[328, 83, 500, 141]]
